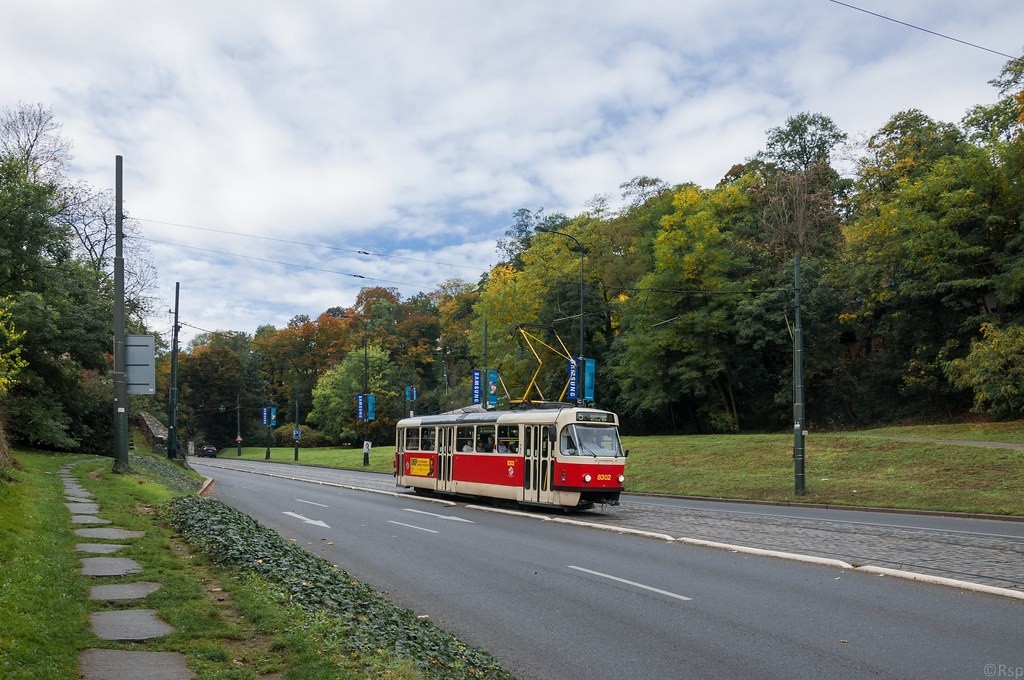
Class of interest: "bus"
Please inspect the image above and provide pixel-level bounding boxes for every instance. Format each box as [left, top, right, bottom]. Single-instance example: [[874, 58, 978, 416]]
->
[[394, 323, 629, 514]]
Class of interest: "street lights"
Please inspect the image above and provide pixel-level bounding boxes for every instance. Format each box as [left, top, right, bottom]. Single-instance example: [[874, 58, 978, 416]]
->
[[534, 226, 586, 407], [340, 315, 369, 466]]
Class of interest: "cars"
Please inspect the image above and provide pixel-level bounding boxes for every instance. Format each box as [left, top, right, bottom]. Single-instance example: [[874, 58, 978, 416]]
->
[[198, 445, 217, 458]]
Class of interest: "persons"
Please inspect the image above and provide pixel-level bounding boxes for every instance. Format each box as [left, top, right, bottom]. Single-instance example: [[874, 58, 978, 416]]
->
[[406, 430, 599, 456], [363, 443, 371, 452]]
